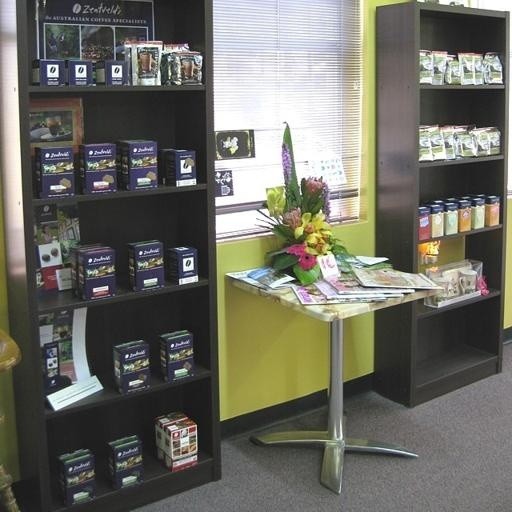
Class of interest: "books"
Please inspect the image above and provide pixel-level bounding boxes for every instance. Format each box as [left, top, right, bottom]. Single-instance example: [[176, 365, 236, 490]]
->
[[225, 252, 443, 315]]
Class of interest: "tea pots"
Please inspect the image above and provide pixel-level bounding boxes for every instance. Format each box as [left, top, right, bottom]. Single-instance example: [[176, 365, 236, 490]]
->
[[432, 275, 460, 307]]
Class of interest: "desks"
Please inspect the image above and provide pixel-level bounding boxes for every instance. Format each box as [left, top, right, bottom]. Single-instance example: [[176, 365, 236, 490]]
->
[[0, 325, 23, 512], [224, 262, 441, 493]]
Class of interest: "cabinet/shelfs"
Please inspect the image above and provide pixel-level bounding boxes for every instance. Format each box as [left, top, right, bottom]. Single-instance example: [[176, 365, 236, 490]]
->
[[0, 0, 222, 512], [374, 2, 510, 410]]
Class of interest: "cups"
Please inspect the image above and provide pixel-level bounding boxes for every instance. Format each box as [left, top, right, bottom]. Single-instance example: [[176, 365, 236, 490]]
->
[[459, 270, 477, 296]]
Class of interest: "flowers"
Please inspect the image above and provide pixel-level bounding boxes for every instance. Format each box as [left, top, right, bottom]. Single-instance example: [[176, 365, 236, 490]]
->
[[255, 121, 349, 285]]
